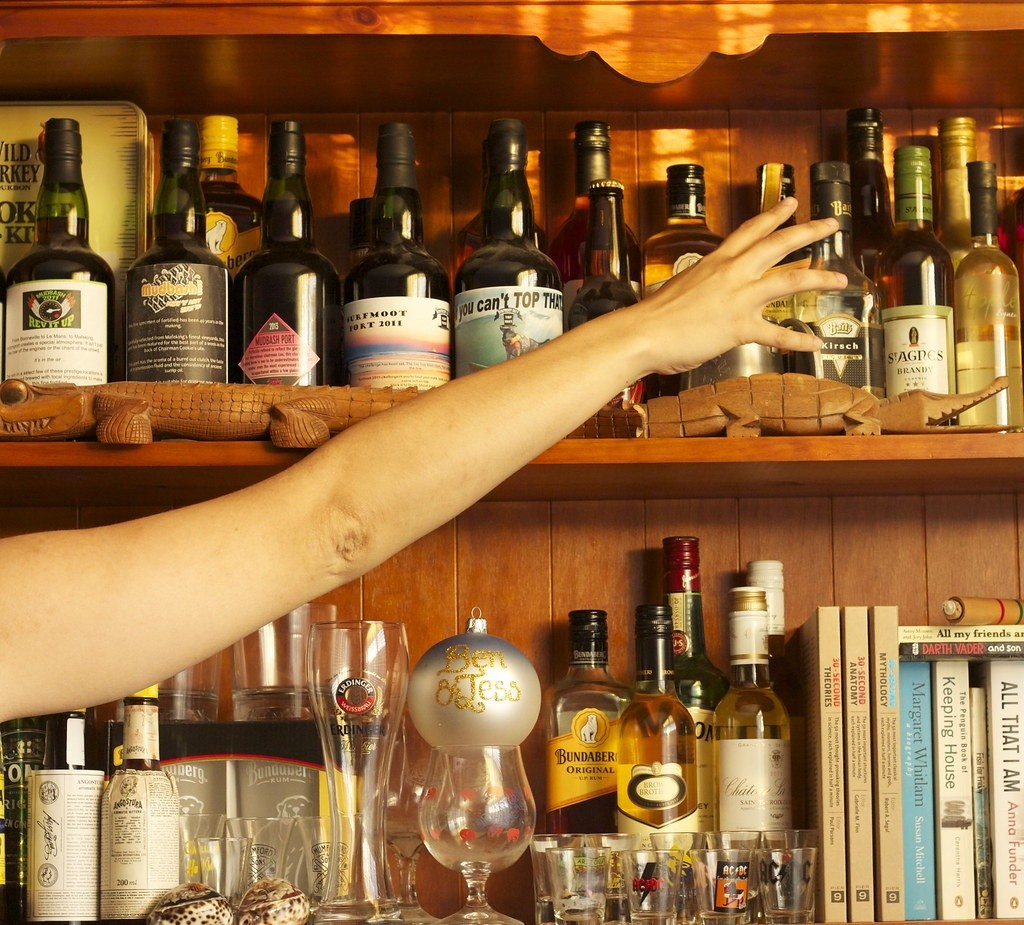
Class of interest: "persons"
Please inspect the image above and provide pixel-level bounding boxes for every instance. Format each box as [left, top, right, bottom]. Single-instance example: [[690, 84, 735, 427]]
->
[[0, 198, 848, 724]]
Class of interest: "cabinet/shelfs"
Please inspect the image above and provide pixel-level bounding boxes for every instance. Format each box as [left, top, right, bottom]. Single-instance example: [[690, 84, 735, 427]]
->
[[0, 0, 1023, 918]]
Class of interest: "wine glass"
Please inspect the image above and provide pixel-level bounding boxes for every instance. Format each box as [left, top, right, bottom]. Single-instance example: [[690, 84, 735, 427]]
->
[[417, 744, 536, 925], [377, 696, 444, 925]]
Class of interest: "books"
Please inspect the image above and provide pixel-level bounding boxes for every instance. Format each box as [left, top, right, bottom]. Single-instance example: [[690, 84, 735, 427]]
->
[[800, 605, 1024, 923]]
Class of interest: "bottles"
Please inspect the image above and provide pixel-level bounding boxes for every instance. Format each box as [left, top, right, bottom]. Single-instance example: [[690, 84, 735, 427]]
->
[[614, 603, 699, 899], [710, 586, 794, 854], [659, 535, 730, 850], [535, 609, 633, 834], [747, 556, 808, 830], [23, 707, 100, 925], [99, 684, 181, 925], [0, 114, 1024, 434]]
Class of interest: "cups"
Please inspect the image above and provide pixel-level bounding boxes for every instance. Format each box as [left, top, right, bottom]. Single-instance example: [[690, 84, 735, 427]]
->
[[529, 829, 819, 925], [303, 619, 405, 924], [181, 813, 362, 925], [115, 645, 223, 724], [231, 599, 337, 722]]
